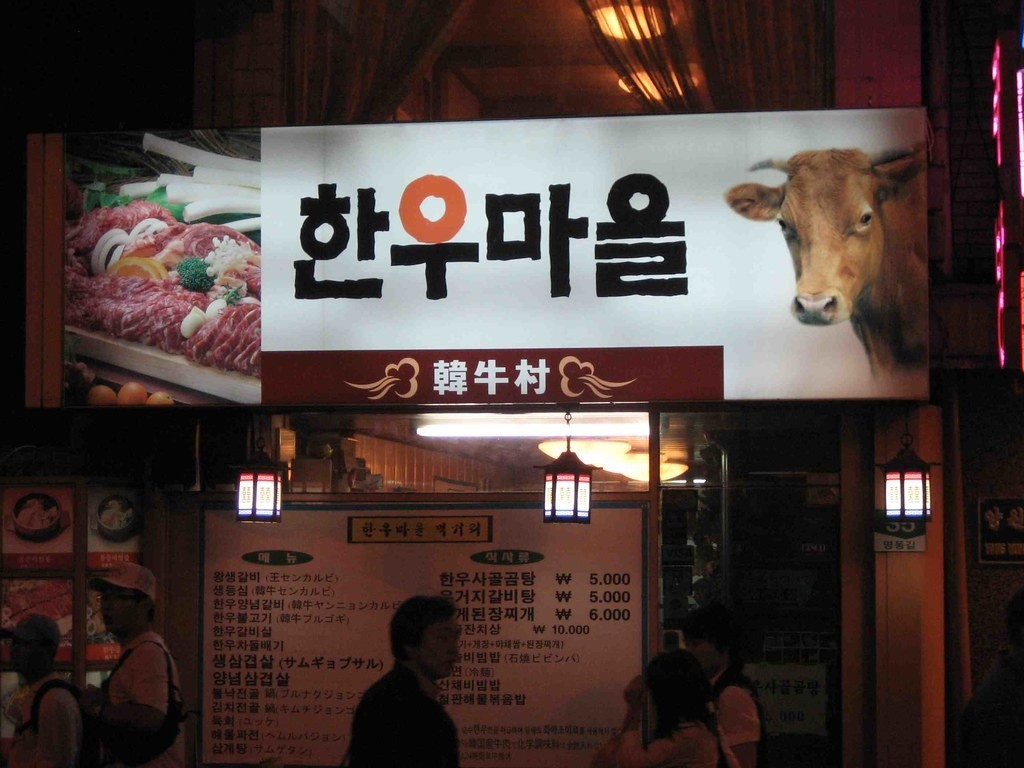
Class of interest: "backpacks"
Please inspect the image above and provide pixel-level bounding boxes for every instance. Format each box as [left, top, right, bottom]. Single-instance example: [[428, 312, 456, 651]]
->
[[25, 678, 97, 768]]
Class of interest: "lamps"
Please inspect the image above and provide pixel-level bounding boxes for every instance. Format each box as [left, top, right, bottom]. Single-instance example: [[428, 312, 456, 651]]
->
[[229, 416, 293, 523], [533, 412, 603, 524], [411, 421, 690, 482], [872, 403, 942, 522], [591, 4, 698, 102]]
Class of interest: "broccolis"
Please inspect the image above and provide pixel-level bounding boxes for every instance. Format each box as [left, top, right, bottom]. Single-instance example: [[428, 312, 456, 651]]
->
[[177, 256, 218, 292]]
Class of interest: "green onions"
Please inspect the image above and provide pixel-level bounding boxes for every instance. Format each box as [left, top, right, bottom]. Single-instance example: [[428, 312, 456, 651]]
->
[[117, 133, 261, 237]]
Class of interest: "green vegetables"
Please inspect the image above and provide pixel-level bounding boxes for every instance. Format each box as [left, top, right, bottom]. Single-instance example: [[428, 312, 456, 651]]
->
[[221, 282, 245, 307]]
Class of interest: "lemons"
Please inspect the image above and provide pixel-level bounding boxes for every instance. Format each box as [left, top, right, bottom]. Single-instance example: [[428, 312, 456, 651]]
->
[[107, 256, 169, 280]]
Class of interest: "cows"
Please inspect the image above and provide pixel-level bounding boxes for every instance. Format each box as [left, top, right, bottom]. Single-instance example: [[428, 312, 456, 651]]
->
[[721, 147, 928, 396]]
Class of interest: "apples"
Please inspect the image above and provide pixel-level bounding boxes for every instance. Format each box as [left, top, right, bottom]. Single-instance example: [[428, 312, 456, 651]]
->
[[88, 382, 174, 405]]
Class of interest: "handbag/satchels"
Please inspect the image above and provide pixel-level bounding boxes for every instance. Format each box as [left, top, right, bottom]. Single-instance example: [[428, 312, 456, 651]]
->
[[96, 641, 184, 767]]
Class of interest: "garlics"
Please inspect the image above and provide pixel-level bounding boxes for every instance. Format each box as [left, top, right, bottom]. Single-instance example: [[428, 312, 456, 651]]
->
[[180, 298, 227, 338]]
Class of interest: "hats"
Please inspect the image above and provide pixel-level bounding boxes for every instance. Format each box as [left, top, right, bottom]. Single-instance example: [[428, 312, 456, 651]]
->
[[0, 613, 62, 653], [86, 559, 159, 602]]
